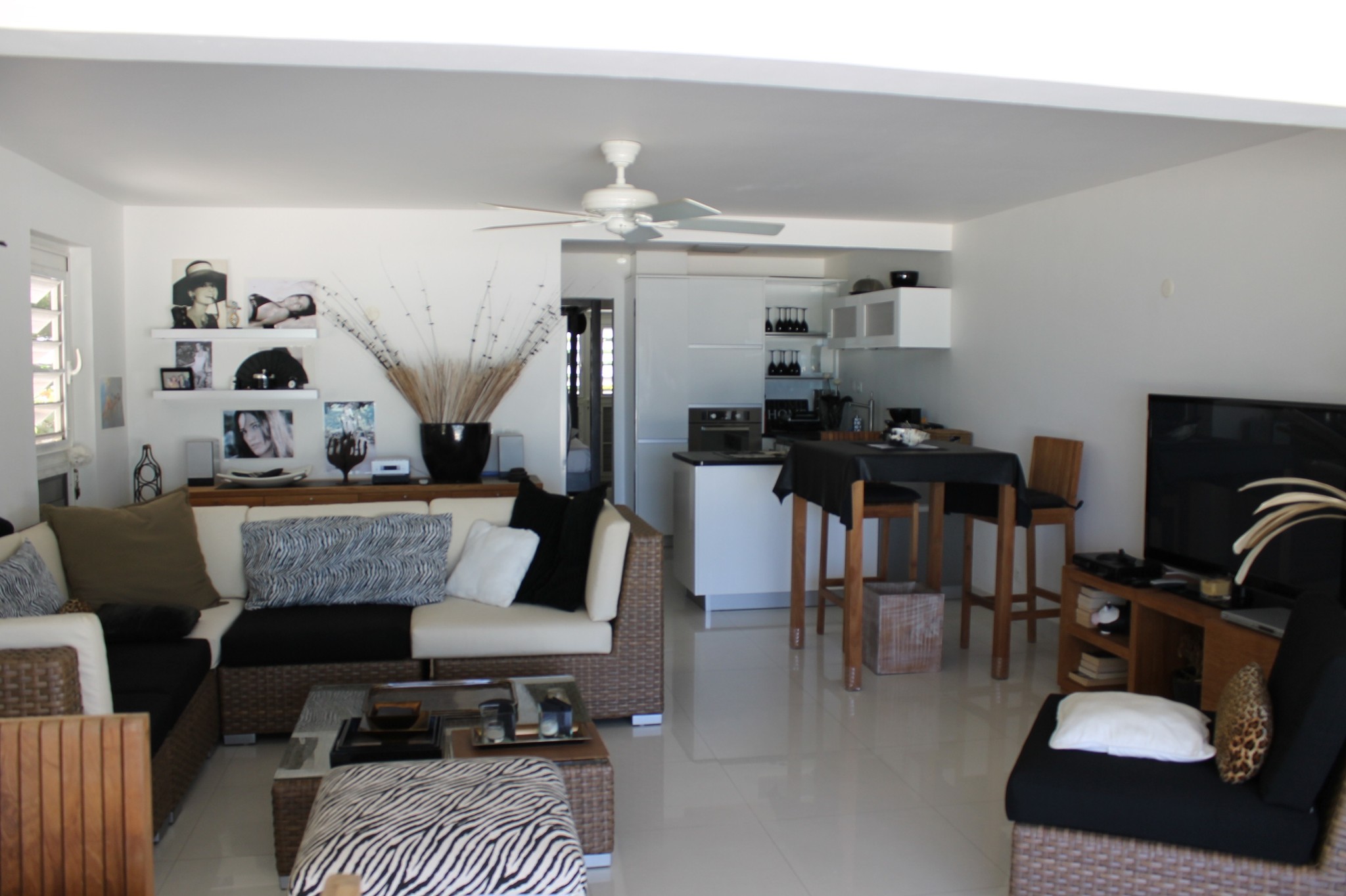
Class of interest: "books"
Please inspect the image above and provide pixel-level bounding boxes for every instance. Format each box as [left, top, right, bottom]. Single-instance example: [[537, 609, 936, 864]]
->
[[1068, 585, 1128, 688]]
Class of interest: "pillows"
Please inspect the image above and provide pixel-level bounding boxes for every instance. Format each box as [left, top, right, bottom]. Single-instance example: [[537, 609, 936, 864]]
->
[[1048, 664, 1277, 784], [239, 513, 453, 610], [444, 521, 539, 608], [41, 485, 221, 612], [509, 477, 607, 612], [97, 604, 200, 642]]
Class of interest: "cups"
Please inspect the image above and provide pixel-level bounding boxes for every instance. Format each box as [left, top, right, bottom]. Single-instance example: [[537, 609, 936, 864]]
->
[[537, 702, 573, 740], [479, 703, 517, 745], [252, 373, 268, 390]]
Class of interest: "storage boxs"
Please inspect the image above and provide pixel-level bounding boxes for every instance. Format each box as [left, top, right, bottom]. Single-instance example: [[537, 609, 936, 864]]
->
[[862, 581, 945, 674], [1201, 579, 1231, 596]]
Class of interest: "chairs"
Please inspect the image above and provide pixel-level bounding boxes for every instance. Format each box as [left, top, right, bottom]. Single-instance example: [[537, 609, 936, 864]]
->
[[1005, 600, 1346, 896]]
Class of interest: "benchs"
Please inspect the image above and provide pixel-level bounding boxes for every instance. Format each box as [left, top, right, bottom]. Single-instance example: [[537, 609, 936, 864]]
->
[[288, 757, 588, 896]]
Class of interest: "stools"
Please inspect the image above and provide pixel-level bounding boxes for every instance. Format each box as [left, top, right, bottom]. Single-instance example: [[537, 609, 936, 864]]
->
[[818, 432, 1082, 649]]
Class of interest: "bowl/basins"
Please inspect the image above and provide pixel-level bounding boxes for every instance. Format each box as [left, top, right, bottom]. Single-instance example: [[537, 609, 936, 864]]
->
[[889, 270, 918, 288]]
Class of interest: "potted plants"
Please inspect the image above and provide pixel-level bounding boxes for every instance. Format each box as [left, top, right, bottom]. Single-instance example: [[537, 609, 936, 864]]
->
[[419, 422, 492, 480]]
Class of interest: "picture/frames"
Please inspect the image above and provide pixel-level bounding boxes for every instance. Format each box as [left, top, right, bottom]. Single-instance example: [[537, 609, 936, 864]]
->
[[160, 367, 196, 390]]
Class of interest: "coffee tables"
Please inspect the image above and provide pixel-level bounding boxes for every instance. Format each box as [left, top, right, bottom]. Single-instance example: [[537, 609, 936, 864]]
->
[[270, 674, 614, 891]]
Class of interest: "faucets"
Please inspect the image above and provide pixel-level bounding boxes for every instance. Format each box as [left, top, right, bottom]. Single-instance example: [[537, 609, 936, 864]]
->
[[848, 391, 874, 431]]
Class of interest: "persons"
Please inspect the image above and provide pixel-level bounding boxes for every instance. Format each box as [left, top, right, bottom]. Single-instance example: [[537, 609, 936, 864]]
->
[[167, 342, 213, 392], [173, 261, 226, 328], [246, 293, 317, 329], [226, 410, 295, 458]]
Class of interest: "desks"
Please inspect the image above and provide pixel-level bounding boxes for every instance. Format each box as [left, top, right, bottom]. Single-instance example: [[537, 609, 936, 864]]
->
[[772, 438, 1033, 690], [183, 475, 542, 507]]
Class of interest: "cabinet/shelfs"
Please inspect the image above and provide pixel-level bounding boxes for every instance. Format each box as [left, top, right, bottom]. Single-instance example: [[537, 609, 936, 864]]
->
[[761, 275, 951, 379], [1058, 562, 1280, 712], [151, 328, 319, 400]]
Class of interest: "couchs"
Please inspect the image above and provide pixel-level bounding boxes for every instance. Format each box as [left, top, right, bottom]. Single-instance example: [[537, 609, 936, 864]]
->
[[0, 497, 662, 896]]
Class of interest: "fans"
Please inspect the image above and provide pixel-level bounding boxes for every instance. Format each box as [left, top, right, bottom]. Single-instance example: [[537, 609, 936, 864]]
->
[[473, 141, 786, 243]]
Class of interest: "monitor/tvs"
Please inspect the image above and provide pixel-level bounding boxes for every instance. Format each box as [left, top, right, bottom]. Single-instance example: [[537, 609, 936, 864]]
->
[[1138, 394, 1346, 603]]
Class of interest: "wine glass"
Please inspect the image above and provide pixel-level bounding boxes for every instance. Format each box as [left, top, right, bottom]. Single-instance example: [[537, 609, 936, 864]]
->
[[765, 306, 773, 332], [768, 349, 801, 376], [775, 306, 809, 332]]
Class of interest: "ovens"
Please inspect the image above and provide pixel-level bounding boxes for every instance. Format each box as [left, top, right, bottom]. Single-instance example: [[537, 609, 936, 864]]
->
[[688, 403, 762, 451]]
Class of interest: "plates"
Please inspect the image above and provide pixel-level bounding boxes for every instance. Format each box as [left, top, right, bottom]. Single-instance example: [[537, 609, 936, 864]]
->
[[216, 467, 312, 488]]
